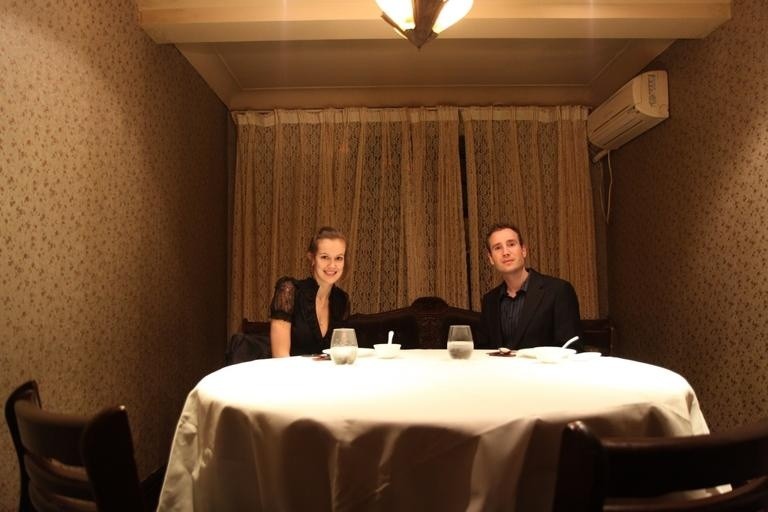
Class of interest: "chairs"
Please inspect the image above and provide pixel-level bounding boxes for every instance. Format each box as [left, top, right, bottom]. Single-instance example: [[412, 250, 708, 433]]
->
[[5, 381, 167, 512], [552, 419, 768, 512]]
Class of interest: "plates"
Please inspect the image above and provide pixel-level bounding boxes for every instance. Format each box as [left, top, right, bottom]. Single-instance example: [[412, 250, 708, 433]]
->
[[517, 344, 577, 361], [324, 348, 375, 356]]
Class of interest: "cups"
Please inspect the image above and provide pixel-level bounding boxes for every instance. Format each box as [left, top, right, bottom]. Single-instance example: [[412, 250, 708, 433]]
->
[[330, 328, 358, 364], [446, 324, 475, 360]]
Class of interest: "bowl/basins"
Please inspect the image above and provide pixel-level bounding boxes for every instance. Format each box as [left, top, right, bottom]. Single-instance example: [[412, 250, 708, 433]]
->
[[373, 341, 401, 358], [576, 351, 602, 368]]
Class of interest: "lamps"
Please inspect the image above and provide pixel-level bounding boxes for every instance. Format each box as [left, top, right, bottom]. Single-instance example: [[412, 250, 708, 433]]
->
[[375, 0, 473, 51]]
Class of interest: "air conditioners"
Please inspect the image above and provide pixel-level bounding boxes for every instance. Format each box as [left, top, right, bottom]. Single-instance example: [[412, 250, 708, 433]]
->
[[587, 70, 670, 151]]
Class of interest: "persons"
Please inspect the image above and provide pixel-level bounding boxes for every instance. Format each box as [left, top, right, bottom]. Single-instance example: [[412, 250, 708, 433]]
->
[[269, 225, 351, 357], [481, 223, 584, 354]]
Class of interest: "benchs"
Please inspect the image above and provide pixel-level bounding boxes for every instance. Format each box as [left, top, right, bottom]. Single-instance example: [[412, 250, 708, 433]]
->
[[225, 296, 613, 368]]
